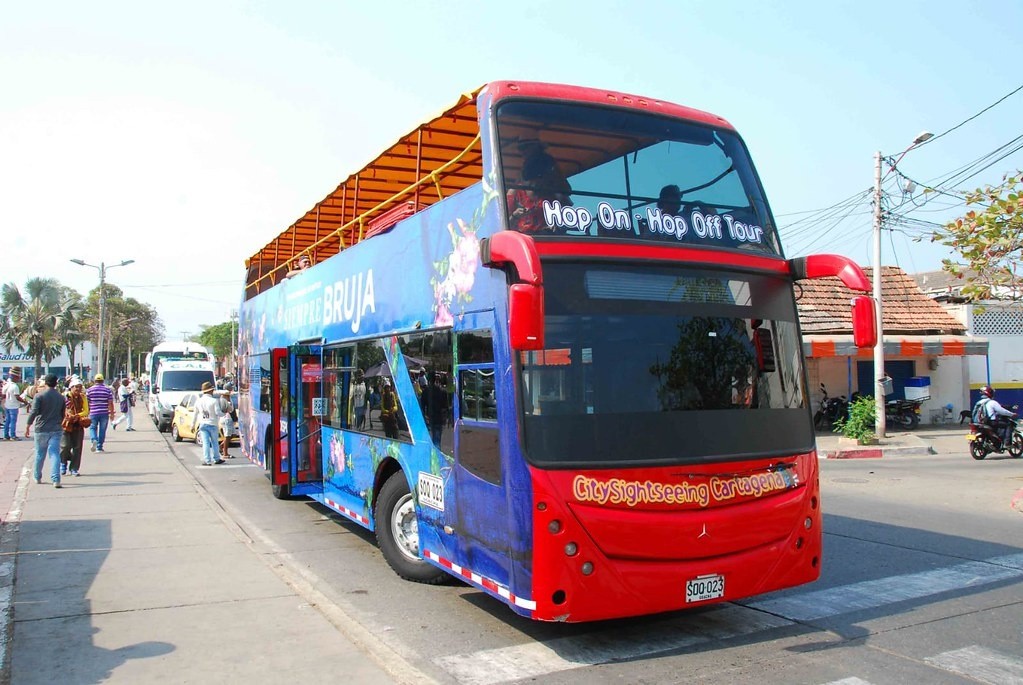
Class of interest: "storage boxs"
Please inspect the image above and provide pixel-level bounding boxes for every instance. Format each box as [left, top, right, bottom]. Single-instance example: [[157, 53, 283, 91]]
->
[[904, 376, 932, 400]]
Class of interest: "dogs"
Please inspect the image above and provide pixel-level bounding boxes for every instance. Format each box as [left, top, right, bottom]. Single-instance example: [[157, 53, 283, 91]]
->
[[958, 410, 973, 429]]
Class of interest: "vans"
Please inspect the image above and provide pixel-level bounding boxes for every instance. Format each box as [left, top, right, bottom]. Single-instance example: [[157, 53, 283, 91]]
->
[[151, 358, 218, 431]]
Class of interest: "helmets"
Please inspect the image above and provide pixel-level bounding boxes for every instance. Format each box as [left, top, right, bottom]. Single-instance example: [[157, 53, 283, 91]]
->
[[94, 373, 104, 380], [979, 386, 994, 398]]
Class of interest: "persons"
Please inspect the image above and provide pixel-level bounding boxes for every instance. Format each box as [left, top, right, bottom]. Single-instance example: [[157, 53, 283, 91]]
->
[[190, 382, 237, 466], [24, 373, 65, 487], [0, 366, 150, 453], [972, 386, 1018, 448], [353, 367, 496, 452], [507, 132, 573, 236], [60, 379, 89, 476], [643, 184, 718, 245], [216, 376, 238, 392]]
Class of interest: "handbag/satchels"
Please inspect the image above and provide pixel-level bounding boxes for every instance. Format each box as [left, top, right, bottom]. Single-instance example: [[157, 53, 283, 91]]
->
[[120, 398, 128, 412]]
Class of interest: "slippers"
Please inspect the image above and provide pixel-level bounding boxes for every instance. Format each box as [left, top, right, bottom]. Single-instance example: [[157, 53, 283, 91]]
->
[[224, 455, 235, 459], [218, 446, 224, 455]]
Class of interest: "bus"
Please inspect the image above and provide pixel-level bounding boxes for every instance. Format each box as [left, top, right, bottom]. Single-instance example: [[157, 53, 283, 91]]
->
[[239, 79, 877, 624], [142, 341, 216, 416]]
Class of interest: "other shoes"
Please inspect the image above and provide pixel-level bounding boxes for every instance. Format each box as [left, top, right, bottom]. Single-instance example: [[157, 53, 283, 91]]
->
[[90, 440, 98, 452], [32, 473, 41, 483], [1003, 444, 1015, 448], [98, 449, 104, 452], [201, 462, 211, 466], [3, 437, 10, 441], [126, 428, 135, 431], [10, 437, 22, 440], [54, 482, 61, 487], [60, 463, 67, 474], [0, 424, 5, 427], [71, 469, 78, 476], [216, 459, 226, 464], [110, 422, 117, 430]]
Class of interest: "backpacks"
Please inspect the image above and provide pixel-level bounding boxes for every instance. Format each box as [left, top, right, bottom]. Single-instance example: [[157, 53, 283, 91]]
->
[[972, 399, 996, 424]]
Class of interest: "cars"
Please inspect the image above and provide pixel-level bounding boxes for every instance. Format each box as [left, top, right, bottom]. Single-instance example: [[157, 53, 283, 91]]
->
[[171, 392, 241, 448]]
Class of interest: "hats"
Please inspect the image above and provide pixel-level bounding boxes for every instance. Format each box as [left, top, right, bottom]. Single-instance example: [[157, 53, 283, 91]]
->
[[220, 389, 230, 396], [420, 367, 426, 374], [430, 371, 440, 380], [122, 378, 131, 383], [201, 382, 216, 392], [383, 381, 390, 387], [64, 374, 83, 388]]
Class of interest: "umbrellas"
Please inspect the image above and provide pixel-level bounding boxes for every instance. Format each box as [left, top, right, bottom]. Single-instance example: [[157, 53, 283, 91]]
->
[[361, 354, 429, 378]]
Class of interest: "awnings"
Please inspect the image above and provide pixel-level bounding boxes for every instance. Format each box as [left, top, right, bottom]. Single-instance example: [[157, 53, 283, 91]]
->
[[802, 335, 990, 421]]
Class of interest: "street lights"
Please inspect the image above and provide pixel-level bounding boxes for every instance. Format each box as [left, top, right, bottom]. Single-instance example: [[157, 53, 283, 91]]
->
[[84, 313, 137, 380], [873, 131, 934, 442], [69, 258, 135, 374]]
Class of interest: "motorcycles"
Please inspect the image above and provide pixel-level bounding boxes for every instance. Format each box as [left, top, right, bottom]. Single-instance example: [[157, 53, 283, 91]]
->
[[814, 382, 862, 432], [964, 404, 1023, 460], [868, 395, 931, 430]]
